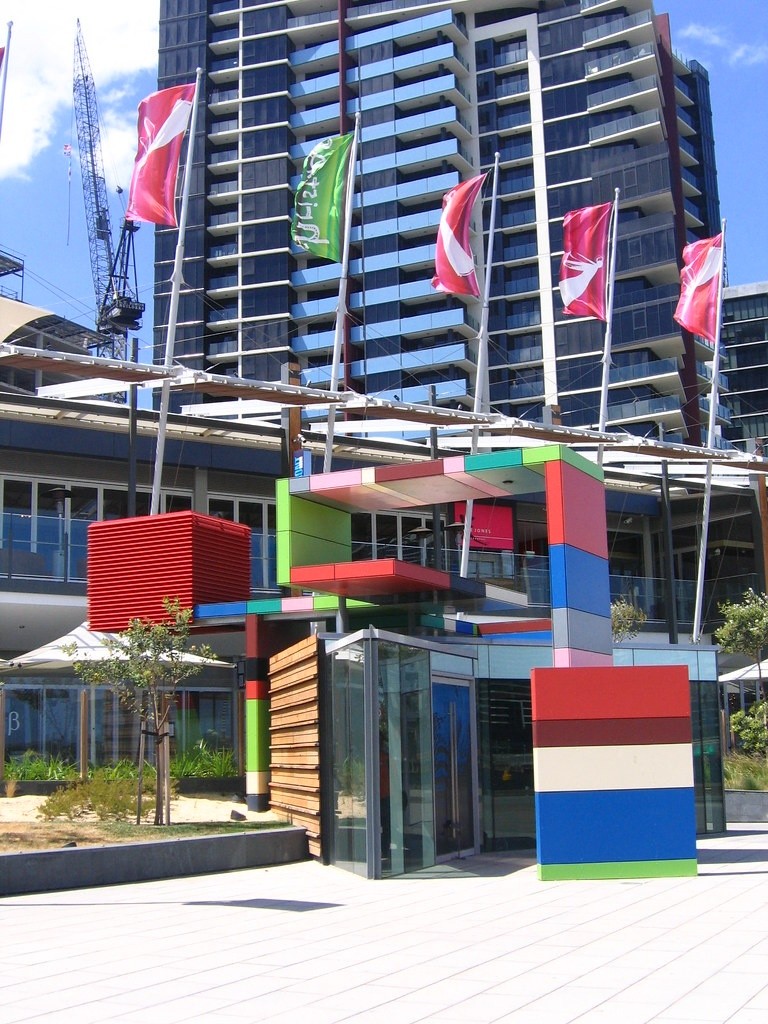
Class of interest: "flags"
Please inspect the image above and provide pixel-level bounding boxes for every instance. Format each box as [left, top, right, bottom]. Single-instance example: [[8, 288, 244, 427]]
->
[[125, 83, 194, 229], [290, 133, 352, 263], [557, 202, 607, 321], [431, 173, 481, 296], [673, 235, 720, 342]]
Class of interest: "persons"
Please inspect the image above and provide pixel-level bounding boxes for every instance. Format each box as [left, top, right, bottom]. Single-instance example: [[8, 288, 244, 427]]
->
[[374, 730, 408, 870]]
